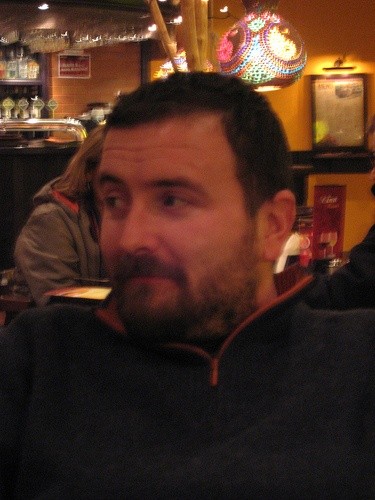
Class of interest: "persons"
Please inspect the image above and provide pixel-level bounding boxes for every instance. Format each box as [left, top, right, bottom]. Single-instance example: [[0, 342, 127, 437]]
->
[[301, 165, 375, 311], [15, 125, 110, 307], [0, 72, 375, 500]]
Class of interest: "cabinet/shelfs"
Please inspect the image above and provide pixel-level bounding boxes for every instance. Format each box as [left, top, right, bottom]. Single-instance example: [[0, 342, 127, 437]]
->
[[0, 40, 54, 140]]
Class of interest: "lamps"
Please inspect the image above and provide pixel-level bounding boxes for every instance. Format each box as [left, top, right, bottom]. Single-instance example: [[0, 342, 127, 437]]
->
[[160, 51, 214, 80], [218, 0, 308, 92]]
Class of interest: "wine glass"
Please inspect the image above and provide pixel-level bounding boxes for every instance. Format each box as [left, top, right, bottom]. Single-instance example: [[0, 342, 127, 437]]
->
[[0, 16, 156, 52], [316, 232, 339, 259]]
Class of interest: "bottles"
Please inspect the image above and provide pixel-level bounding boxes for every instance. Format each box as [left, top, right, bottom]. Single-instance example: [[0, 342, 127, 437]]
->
[[0, 46, 42, 120], [76, 107, 114, 133], [293, 205, 313, 262]]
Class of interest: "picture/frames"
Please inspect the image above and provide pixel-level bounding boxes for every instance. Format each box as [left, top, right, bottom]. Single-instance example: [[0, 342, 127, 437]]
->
[[309, 74, 368, 152]]
